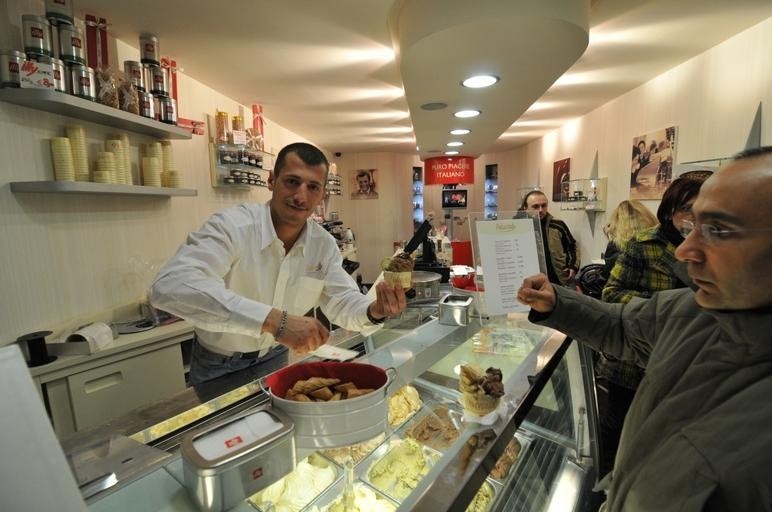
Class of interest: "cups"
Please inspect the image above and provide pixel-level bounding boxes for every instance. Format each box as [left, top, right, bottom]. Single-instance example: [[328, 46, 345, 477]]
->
[[51, 125, 184, 189], [384, 269, 412, 290]]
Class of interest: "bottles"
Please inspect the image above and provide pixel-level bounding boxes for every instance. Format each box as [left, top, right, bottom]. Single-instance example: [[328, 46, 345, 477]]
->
[[346, 227, 353, 249], [215, 112, 230, 144], [217, 149, 268, 186], [252, 103, 264, 150], [232, 117, 246, 149], [331, 211, 340, 221]]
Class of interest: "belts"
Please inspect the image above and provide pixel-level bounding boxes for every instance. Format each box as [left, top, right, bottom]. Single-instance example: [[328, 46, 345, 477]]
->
[[193, 331, 274, 360]]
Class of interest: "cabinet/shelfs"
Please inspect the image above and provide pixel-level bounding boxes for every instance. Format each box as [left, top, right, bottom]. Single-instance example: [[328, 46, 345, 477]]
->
[[28, 311, 197, 440], [558, 177, 609, 213], [1, 85, 201, 197], [208, 140, 278, 191], [57, 298, 606, 512]]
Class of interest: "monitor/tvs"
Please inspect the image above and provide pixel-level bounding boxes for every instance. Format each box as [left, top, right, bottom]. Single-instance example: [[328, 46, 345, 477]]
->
[[441, 189, 467, 209], [403, 219, 442, 266]]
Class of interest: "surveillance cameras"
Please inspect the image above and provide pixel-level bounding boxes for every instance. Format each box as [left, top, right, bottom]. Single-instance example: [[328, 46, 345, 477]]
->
[[336, 153, 342, 158]]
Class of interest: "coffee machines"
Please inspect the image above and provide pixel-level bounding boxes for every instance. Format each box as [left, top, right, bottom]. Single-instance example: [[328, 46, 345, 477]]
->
[[322, 221, 343, 250]]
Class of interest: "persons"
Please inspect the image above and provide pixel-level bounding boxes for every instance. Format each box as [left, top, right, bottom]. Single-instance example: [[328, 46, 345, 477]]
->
[[596, 170, 713, 481], [146, 142, 409, 388], [583, 198, 661, 299], [630, 139, 672, 187], [512, 189, 582, 286], [350, 171, 380, 200], [516, 144, 772, 511]]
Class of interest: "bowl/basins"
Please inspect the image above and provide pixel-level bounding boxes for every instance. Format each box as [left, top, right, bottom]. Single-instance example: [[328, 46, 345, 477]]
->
[[459, 387, 500, 416], [258, 361, 398, 449]]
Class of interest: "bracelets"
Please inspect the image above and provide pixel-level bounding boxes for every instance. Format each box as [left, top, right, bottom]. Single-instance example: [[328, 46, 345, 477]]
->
[[272, 309, 289, 343], [366, 305, 388, 326]]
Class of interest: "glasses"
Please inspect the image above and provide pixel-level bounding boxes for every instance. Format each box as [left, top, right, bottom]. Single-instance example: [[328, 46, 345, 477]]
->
[[680, 213, 772, 245]]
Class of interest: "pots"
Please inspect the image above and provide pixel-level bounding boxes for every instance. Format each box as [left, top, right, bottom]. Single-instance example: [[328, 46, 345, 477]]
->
[[407, 271, 442, 301]]
